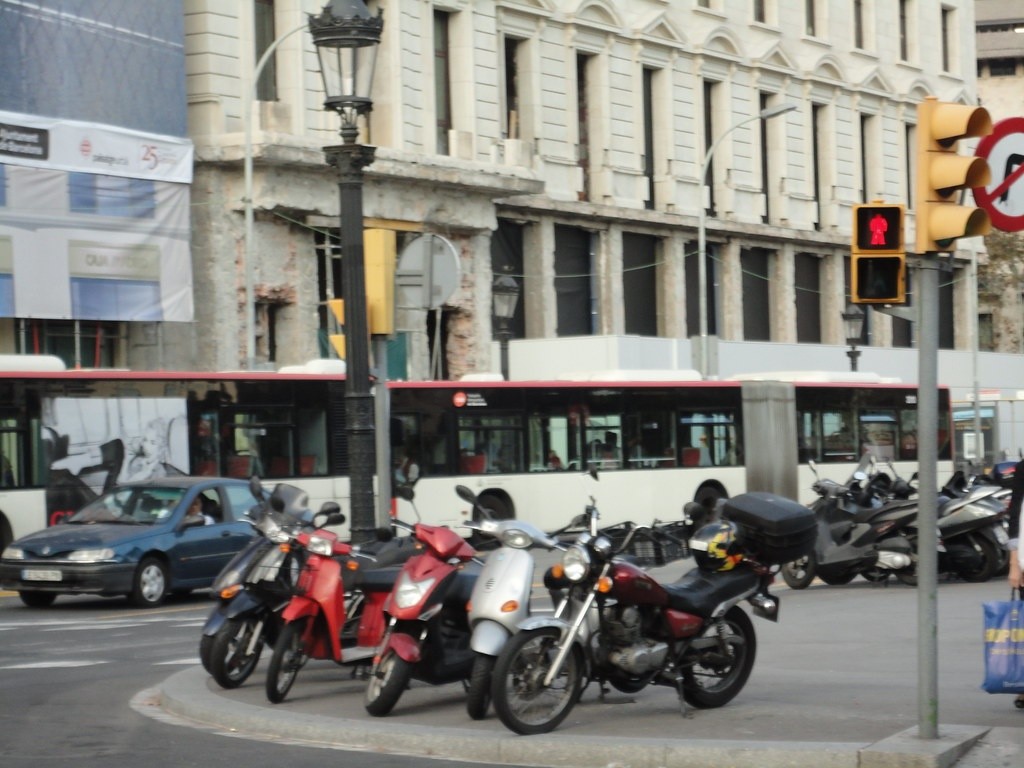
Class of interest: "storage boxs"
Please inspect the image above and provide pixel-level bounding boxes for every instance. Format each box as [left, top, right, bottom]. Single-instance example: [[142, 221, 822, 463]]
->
[[993, 461, 1019, 488], [721, 491, 819, 565]]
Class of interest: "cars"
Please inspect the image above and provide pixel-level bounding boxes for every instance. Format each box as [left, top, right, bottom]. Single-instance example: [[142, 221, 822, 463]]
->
[[0, 475, 313, 610]]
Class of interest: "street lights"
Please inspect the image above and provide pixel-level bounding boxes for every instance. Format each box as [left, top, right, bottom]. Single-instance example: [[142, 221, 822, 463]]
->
[[306, 0, 387, 547], [841, 304, 868, 371], [491, 266, 519, 381], [698, 103, 798, 383]]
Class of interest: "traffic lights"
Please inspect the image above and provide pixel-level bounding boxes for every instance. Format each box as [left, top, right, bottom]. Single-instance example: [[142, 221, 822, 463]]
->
[[324, 299, 347, 359], [852, 204, 906, 310], [914, 99, 993, 256]]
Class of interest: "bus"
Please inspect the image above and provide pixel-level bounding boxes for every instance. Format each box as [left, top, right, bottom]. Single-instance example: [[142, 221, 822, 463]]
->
[[0, 353, 956, 594]]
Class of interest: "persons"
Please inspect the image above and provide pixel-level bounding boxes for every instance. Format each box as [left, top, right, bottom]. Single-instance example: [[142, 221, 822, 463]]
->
[[158, 496, 214, 525], [46, 418, 167, 525], [1008, 459, 1024, 708], [393, 444, 419, 482], [475, 429, 501, 466]]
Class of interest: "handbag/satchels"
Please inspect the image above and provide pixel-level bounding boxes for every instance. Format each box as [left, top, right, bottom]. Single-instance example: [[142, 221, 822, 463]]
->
[[980, 586, 1023, 696]]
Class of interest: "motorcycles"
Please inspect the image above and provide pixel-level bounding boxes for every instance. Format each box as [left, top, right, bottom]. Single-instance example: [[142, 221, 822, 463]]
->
[[782, 445, 1019, 588], [198, 457, 819, 736]]
